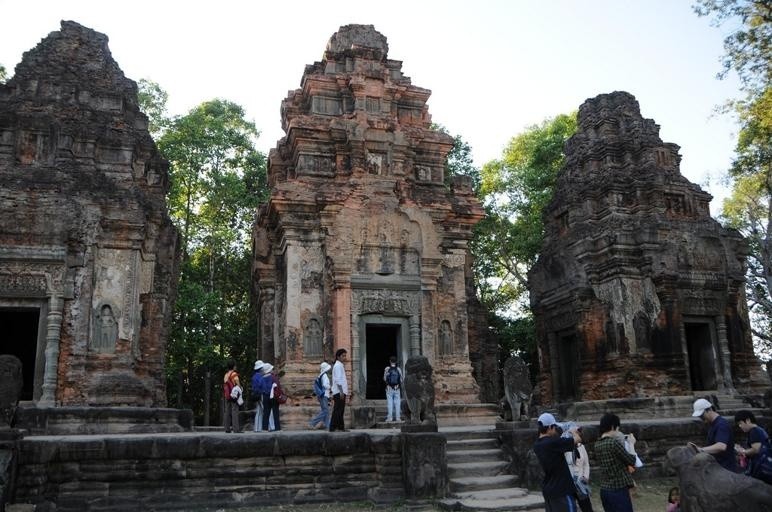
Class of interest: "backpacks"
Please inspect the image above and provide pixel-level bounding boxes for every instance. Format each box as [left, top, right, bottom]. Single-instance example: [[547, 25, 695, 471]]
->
[[387, 366, 399, 385], [314, 372, 326, 397], [748, 428, 772, 482]]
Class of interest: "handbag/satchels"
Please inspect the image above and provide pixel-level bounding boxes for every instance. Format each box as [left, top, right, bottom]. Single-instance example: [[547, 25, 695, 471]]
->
[[575, 475, 591, 500], [275, 389, 286, 403], [223, 379, 234, 399], [248, 391, 260, 400]]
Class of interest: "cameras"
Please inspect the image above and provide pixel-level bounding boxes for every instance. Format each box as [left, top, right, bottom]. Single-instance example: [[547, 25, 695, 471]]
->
[[622, 434, 629, 440]]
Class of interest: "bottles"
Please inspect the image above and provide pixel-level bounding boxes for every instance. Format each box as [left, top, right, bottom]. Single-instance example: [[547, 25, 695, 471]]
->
[[738, 452, 746, 468]]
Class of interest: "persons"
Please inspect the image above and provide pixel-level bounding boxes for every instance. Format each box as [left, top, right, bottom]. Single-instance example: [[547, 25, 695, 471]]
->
[[535, 397, 772, 511], [440, 322, 454, 359], [305, 319, 322, 354], [97, 306, 116, 350], [382, 355, 404, 423], [224, 361, 242, 432], [250, 349, 351, 432]]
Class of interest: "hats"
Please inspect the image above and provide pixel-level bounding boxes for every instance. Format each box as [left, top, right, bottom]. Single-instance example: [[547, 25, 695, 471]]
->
[[320, 362, 331, 371], [561, 421, 580, 439], [692, 399, 711, 417], [264, 363, 273, 373], [253, 360, 263, 370], [538, 412, 561, 426]]
